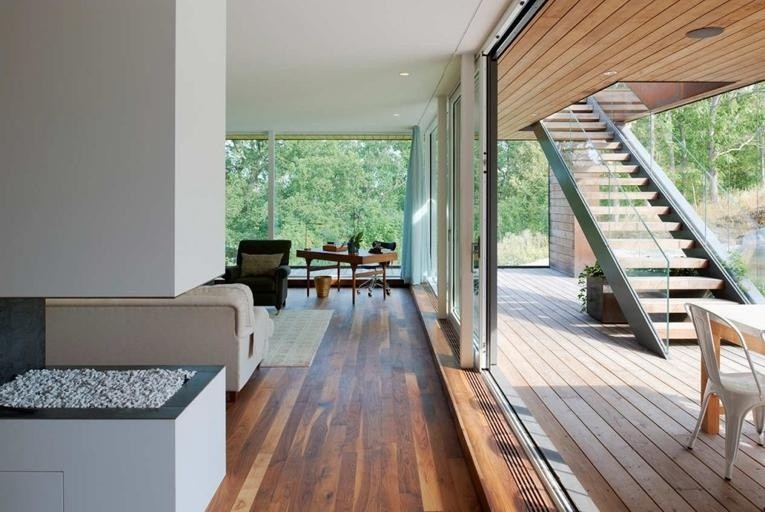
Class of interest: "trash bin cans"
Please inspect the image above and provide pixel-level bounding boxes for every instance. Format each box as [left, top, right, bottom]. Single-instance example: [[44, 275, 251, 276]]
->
[[314, 275, 332, 298]]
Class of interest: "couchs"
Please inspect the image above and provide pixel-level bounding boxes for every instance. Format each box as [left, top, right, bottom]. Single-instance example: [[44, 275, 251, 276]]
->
[[45, 284, 271, 403]]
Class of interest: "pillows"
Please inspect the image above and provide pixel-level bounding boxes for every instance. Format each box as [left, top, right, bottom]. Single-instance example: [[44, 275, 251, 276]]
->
[[241, 253, 284, 278]]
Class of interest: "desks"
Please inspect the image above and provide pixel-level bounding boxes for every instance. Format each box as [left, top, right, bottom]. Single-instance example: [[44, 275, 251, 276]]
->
[[693, 303, 765, 436], [296, 248, 398, 305]]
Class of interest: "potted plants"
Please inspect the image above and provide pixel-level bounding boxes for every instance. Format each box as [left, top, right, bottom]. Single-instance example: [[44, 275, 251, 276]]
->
[[578, 257, 716, 323], [347, 232, 363, 254]]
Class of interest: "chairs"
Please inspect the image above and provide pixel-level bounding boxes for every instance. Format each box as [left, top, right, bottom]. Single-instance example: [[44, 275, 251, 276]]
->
[[683, 302, 765, 480], [214, 240, 291, 315], [356, 241, 396, 296]]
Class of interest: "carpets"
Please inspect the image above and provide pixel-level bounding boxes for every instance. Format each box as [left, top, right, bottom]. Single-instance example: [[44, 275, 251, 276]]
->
[[262, 309, 335, 367]]
